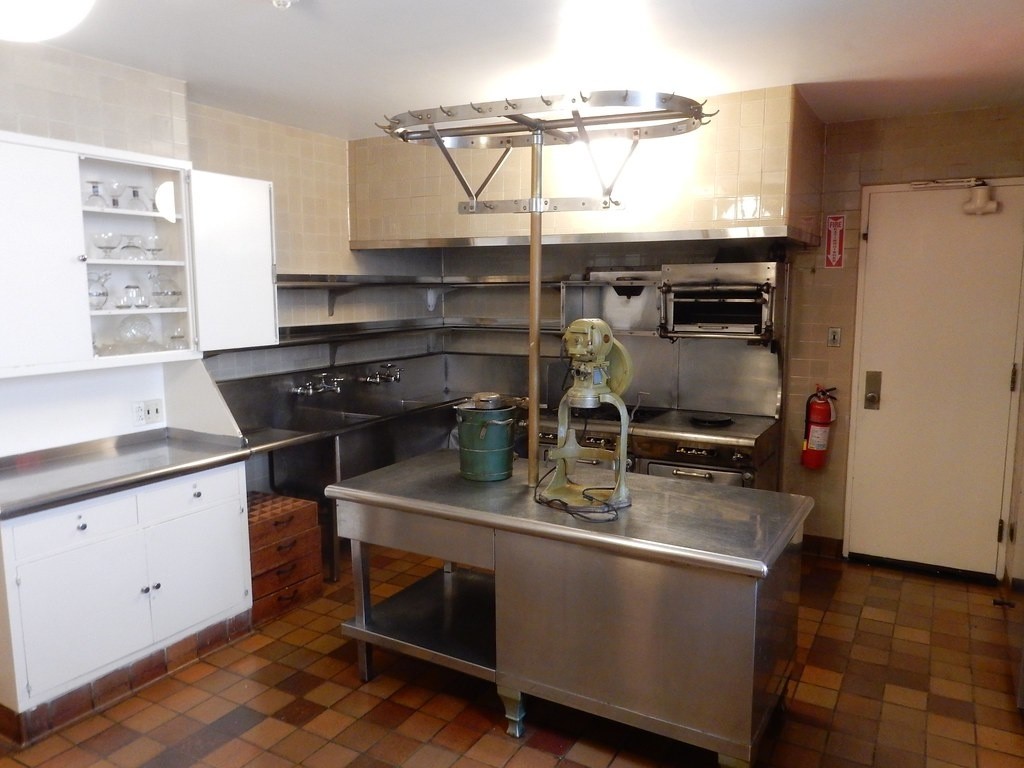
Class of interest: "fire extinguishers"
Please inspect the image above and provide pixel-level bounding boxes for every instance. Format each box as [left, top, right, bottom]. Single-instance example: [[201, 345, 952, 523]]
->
[[802, 383, 838, 471]]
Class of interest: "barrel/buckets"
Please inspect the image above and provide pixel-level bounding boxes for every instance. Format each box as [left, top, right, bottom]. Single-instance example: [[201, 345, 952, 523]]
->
[[455, 405, 516, 482]]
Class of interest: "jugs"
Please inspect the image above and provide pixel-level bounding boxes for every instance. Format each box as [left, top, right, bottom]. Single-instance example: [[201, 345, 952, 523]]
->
[[88, 271, 111, 311]]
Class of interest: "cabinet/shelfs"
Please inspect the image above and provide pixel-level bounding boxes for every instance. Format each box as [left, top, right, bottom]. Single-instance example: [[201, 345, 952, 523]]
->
[[0, 460, 253, 748], [0, 130, 278, 381], [276, 275, 562, 348], [327, 447, 814, 768]]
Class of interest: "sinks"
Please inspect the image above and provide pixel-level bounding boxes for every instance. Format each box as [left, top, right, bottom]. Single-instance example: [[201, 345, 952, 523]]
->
[[272, 407, 378, 435], [349, 399, 427, 417]]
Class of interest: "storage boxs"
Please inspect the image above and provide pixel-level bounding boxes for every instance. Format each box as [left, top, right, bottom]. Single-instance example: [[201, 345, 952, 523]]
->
[[246, 491, 324, 630]]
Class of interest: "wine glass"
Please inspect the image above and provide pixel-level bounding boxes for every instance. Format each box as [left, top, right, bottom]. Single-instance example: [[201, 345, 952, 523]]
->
[[88, 232, 166, 260], [84, 180, 159, 212]]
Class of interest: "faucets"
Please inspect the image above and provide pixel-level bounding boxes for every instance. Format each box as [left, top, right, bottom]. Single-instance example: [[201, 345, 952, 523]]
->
[[317, 372, 345, 393], [380, 363, 404, 384]]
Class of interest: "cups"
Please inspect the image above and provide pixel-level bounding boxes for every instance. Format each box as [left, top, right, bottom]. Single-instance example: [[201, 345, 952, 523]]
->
[[114, 285, 150, 309]]
